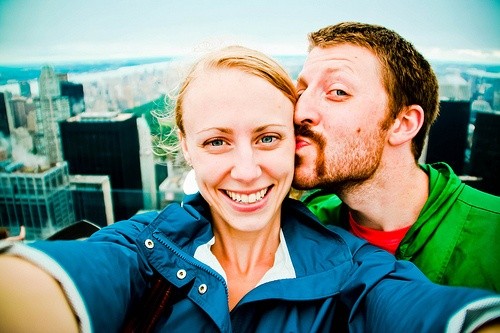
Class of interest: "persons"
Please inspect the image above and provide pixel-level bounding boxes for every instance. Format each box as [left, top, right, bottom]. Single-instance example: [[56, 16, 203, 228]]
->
[[0, 38, 500, 333], [290, 19, 500, 301], [0, 222, 26, 243]]
